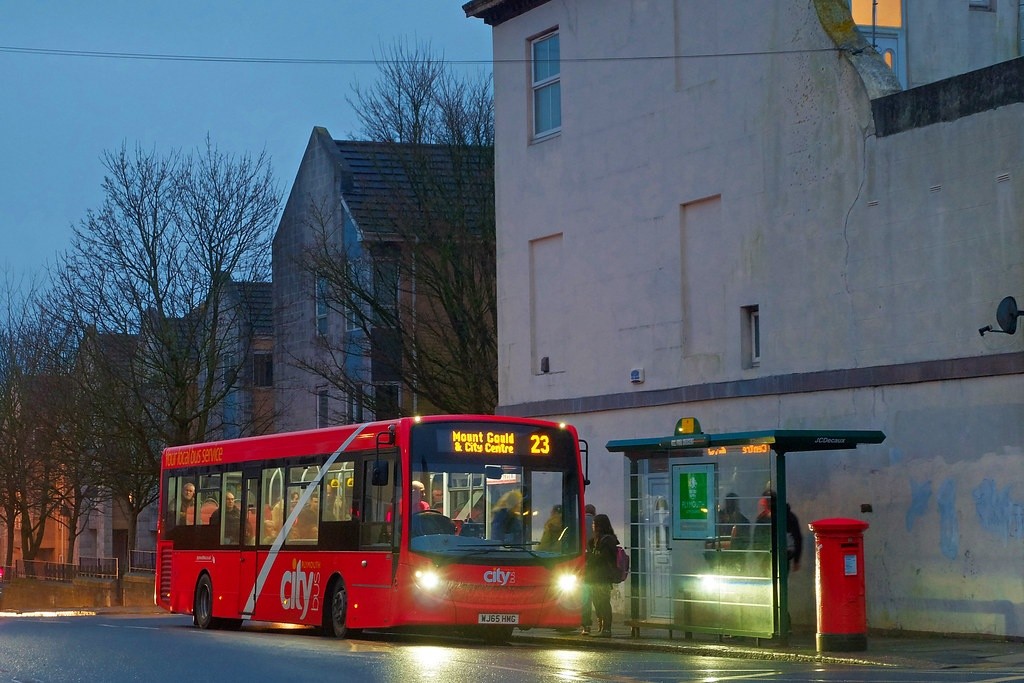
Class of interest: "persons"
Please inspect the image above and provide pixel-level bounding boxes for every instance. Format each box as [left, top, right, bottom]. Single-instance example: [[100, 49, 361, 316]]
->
[[583, 585, 602, 635], [585, 504, 596, 543], [386, 480, 430, 522], [490, 491, 522, 544], [264, 485, 335, 545], [704, 493, 750, 574], [210, 491, 240, 543], [535, 504, 561, 553], [585, 514, 621, 637], [752, 491, 803, 634], [168, 482, 195, 526]]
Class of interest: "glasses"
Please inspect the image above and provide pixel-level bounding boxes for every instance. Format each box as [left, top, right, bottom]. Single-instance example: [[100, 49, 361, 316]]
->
[[311, 501, 318, 503]]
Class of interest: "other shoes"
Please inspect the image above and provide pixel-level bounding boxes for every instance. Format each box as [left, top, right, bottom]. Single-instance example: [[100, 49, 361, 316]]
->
[[582, 626, 591, 634]]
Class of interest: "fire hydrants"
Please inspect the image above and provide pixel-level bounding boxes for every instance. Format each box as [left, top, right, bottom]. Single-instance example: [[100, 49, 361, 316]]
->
[[806, 518, 874, 652]]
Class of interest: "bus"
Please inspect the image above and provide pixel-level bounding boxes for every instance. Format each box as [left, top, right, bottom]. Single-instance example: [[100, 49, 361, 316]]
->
[[154, 415, 593, 636]]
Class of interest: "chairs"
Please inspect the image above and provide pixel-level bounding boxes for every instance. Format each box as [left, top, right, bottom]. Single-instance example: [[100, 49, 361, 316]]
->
[[186, 498, 203, 525], [201, 499, 219, 525], [247, 507, 256, 537]]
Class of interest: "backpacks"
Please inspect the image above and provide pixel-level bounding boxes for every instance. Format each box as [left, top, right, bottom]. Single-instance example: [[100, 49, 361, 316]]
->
[[598, 534, 630, 584]]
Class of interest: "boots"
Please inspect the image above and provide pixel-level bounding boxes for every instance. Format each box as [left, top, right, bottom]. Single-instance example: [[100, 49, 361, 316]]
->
[[593, 618, 611, 638]]
